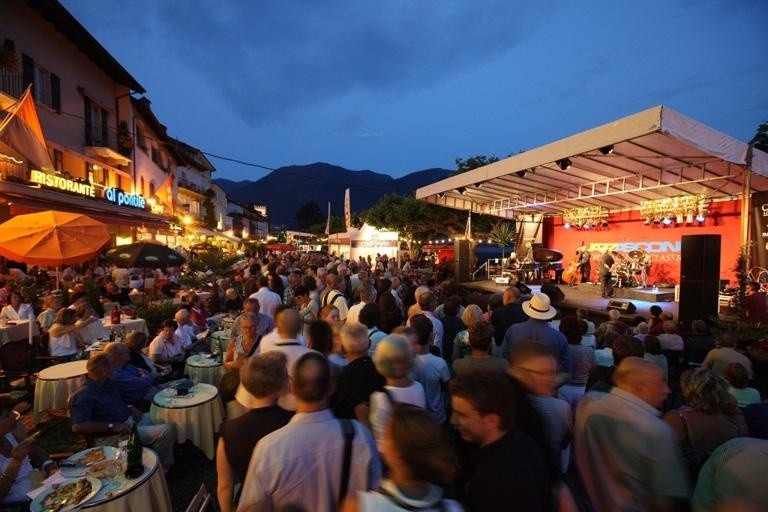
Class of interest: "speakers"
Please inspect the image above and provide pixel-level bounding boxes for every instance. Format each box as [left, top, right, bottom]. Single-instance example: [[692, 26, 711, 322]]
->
[[454, 239, 469, 282], [510, 279, 531, 294], [678, 235, 721, 330], [541, 285, 565, 301]]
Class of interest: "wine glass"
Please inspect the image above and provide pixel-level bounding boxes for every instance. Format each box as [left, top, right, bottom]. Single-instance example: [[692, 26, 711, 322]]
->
[[103, 436, 130, 490], [165, 373, 200, 403]]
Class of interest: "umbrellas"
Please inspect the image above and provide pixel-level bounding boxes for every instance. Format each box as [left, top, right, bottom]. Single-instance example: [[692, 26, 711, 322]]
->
[[109, 242, 184, 292], [0, 209, 113, 267]]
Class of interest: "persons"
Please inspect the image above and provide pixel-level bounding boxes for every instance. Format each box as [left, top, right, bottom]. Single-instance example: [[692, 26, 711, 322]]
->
[[1, 247, 768, 511]]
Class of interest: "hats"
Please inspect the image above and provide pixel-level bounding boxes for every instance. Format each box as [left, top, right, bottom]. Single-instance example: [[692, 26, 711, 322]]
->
[[522, 293, 558, 320]]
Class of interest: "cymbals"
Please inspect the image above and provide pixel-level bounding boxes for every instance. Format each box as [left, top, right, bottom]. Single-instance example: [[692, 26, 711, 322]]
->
[[628, 250, 642, 259]]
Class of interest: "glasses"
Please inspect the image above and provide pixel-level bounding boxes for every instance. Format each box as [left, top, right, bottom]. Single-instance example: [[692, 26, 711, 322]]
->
[[515, 365, 557, 378]]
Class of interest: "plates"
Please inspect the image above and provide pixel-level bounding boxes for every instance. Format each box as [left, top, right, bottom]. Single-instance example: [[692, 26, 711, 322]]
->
[[26, 476, 104, 511], [58, 446, 118, 476]]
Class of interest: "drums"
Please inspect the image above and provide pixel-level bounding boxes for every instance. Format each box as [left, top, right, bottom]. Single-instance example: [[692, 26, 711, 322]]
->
[[631, 263, 639, 270]]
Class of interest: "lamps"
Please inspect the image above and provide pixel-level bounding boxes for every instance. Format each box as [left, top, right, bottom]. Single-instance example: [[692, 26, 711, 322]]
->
[[433, 143, 617, 199], [638, 192, 708, 226], [563, 206, 609, 231]]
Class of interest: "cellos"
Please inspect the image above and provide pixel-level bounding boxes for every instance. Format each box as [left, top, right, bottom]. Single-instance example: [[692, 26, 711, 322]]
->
[[561, 241, 586, 286]]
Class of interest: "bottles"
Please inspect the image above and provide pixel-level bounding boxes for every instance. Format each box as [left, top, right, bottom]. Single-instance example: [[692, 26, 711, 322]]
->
[[211, 336, 223, 363], [105, 307, 137, 324], [127, 420, 145, 479], [98, 325, 126, 342]]
